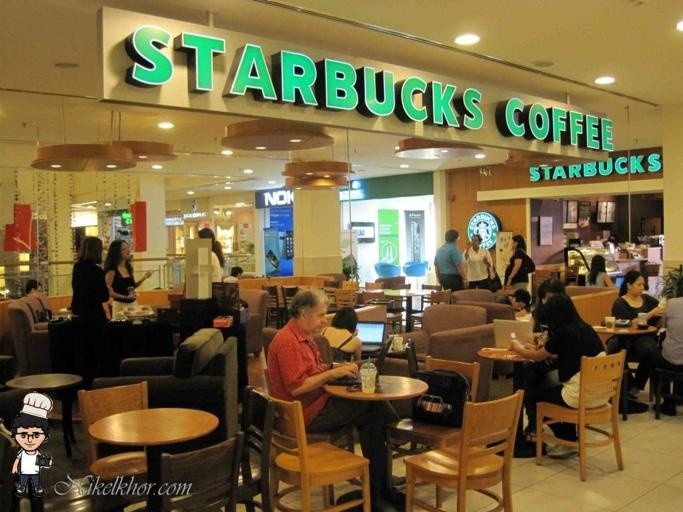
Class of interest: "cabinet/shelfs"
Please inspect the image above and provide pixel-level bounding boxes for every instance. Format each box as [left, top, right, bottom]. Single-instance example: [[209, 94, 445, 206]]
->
[[564, 246, 619, 274]]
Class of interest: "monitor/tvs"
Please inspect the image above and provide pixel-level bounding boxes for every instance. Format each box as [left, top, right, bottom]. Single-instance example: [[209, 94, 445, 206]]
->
[[351, 222, 375, 243]]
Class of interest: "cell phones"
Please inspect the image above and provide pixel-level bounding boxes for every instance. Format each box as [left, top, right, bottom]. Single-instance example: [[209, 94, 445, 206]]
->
[[286, 230, 294, 259], [127, 287, 135, 295], [266, 249, 280, 269]]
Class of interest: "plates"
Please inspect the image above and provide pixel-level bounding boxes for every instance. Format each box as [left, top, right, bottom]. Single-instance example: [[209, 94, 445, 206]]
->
[[637, 324, 649, 329], [593, 327, 607, 330]]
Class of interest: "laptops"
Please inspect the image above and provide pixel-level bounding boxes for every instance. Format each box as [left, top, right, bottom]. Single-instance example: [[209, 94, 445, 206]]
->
[[494, 319, 537, 348], [354, 321, 386, 353], [327, 336, 394, 385], [614, 276, 625, 288]]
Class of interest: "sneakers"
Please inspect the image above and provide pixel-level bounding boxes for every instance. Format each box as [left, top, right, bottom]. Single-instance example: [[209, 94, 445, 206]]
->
[[653, 395, 677, 416], [628, 386, 641, 397], [546, 440, 579, 459], [390, 476, 406, 485]]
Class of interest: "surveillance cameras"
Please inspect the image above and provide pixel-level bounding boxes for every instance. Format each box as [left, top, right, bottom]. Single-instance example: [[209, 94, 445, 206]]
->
[[552, 196, 559, 203]]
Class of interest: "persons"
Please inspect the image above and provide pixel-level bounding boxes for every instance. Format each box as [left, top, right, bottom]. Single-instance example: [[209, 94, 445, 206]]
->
[[521, 278, 566, 444], [509, 293, 611, 460], [103, 238, 157, 319], [25, 277, 43, 297], [263, 289, 407, 488], [67, 235, 113, 321], [603, 268, 667, 400], [461, 233, 497, 290], [651, 278, 682, 417], [195, 225, 225, 285], [582, 255, 616, 286], [433, 229, 467, 294], [222, 264, 244, 285], [321, 305, 363, 366], [493, 288, 531, 313], [503, 234, 537, 295]]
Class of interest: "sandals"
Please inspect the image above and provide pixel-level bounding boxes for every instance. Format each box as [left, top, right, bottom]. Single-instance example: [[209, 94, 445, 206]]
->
[[523, 425, 545, 442]]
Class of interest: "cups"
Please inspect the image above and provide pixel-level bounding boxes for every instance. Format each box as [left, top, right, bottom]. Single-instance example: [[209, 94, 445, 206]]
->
[[621, 248, 626, 253], [603, 231, 610, 239], [596, 236, 600, 240], [606, 317, 616, 333], [638, 313, 648, 325], [393, 336, 403, 351], [359, 363, 378, 396]]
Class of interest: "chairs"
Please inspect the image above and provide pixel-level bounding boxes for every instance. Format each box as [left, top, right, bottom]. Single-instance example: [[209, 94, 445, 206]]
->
[[373, 261, 426, 289]]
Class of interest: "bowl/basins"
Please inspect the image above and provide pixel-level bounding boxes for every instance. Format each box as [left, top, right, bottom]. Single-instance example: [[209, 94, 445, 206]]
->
[[589, 241, 603, 247]]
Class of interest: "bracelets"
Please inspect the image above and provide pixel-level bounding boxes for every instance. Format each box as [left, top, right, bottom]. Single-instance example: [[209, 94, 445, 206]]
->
[[507, 276, 512, 280], [121, 295, 127, 301]]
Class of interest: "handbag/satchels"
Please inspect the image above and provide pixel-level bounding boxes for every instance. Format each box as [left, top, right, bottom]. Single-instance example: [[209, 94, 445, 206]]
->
[[411, 368, 471, 427], [518, 259, 535, 273], [487, 272, 502, 293]]
